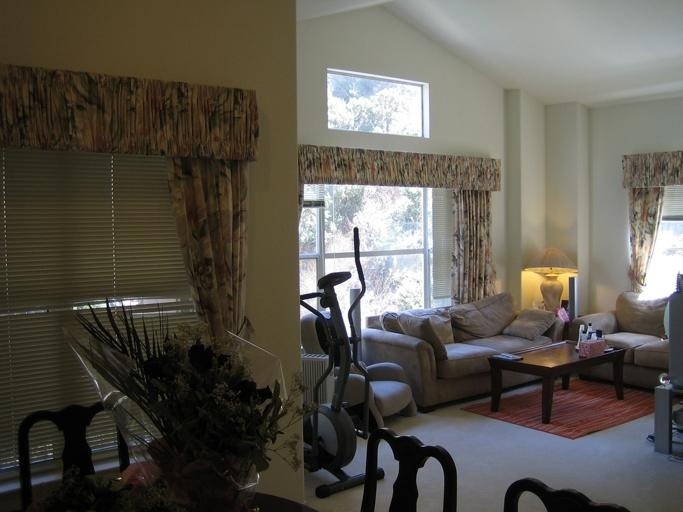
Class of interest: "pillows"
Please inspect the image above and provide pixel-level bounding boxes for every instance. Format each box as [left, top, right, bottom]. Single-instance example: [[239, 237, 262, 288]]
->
[[615, 292, 669, 340], [502, 307, 555, 340], [396, 311, 448, 360], [449, 291, 520, 343], [379, 311, 408, 334]]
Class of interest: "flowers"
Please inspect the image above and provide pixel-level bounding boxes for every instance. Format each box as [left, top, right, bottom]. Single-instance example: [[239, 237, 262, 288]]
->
[[60, 302, 305, 487]]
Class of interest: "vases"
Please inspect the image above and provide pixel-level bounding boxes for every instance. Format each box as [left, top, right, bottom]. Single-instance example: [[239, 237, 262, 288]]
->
[[176, 462, 260, 512]]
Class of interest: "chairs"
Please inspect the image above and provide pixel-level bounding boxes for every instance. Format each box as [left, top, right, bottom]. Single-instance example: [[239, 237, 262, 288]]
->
[[18, 401, 131, 511], [360, 427, 457, 512], [503, 477, 631, 512], [301, 311, 417, 433]]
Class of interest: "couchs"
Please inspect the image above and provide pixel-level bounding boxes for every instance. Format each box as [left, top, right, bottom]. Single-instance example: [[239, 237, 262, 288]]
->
[[361, 305, 564, 413], [568, 309, 670, 389]]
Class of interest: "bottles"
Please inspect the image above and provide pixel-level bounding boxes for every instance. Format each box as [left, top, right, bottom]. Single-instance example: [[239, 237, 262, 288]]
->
[[577, 323, 593, 357]]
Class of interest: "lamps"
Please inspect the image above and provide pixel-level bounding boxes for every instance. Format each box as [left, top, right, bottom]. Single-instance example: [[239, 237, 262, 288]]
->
[[521, 248, 579, 317]]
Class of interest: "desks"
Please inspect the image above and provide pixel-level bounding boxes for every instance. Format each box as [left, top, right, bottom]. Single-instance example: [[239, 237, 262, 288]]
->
[[654, 385, 683, 454], [1, 463, 324, 511]]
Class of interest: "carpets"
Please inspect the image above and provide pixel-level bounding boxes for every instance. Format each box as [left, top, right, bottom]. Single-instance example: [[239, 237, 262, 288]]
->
[[460, 379, 683, 440]]
